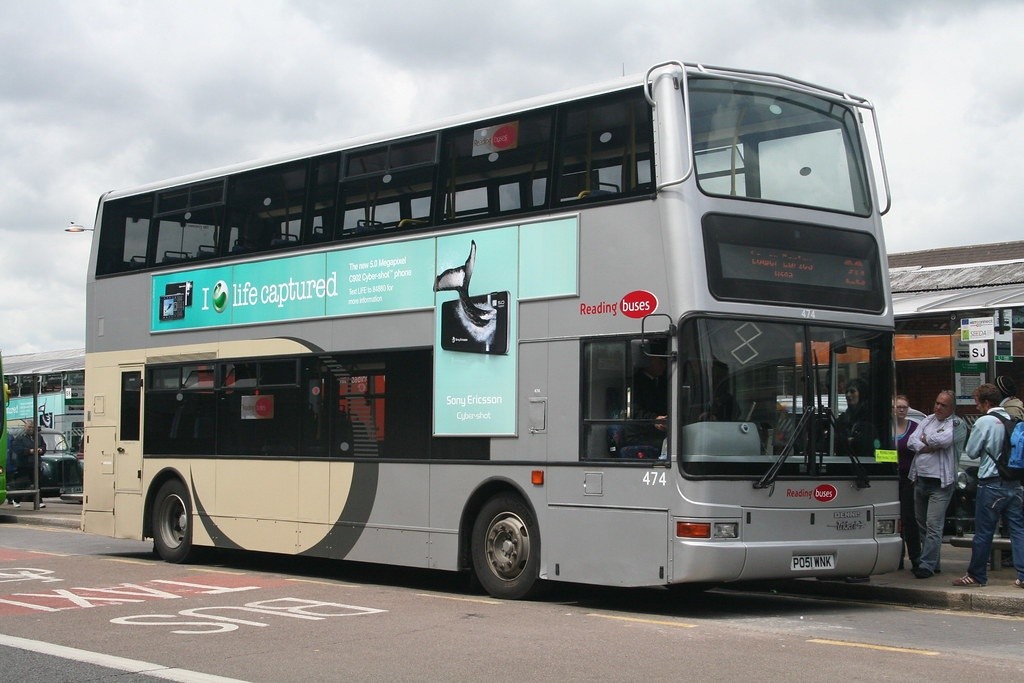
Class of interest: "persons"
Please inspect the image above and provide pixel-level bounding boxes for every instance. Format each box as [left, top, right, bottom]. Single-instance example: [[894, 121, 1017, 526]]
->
[[836, 377, 870, 446], [952, 376, 1024, 589], [620, 357, 668, 455], [7, 419, 46, 508], [892, 390, 968, 577]]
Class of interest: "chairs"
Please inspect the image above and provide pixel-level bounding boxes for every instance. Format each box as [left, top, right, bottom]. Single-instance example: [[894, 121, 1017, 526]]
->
[[130, 190, 620, 267]]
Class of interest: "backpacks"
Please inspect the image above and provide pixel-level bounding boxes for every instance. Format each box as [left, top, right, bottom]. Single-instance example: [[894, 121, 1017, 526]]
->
[[983, 411, 1024, 481]]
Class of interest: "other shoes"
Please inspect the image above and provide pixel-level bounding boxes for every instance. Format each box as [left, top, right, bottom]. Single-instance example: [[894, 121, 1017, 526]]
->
[[12, 499, 21, 508], [32, 502, 46, 508], [911, 567, 941, 579]]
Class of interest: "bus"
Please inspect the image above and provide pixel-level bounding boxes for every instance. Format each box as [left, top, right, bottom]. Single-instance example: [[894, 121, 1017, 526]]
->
[[82, 60, 903, 600], [0, 352, 11, 505], [125, 383, 302, 454]]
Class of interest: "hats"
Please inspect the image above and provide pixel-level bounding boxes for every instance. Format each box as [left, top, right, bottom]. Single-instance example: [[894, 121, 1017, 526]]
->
[[995, 375, 1017, 398]]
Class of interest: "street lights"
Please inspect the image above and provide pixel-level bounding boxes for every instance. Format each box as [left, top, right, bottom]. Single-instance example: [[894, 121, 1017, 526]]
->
[[64, 225, 93, 233]]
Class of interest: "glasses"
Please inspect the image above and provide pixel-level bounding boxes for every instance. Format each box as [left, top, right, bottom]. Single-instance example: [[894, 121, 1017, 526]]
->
[[28, 425, 34, 428], [893, 405, 908, 411]]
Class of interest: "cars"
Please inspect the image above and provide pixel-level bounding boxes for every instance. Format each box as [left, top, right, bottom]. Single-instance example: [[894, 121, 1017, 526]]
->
[[777, 395, 981, 526], [7, 427, 84, 496]]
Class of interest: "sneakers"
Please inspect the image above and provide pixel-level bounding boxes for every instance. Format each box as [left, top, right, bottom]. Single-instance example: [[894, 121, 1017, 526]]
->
[[951, 574, 984, 587], [1013, 578, 1024, 588]]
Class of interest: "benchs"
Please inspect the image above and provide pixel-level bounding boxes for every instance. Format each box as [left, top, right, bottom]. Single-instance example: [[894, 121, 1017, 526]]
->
[[950, 535, 1013, 570]]
